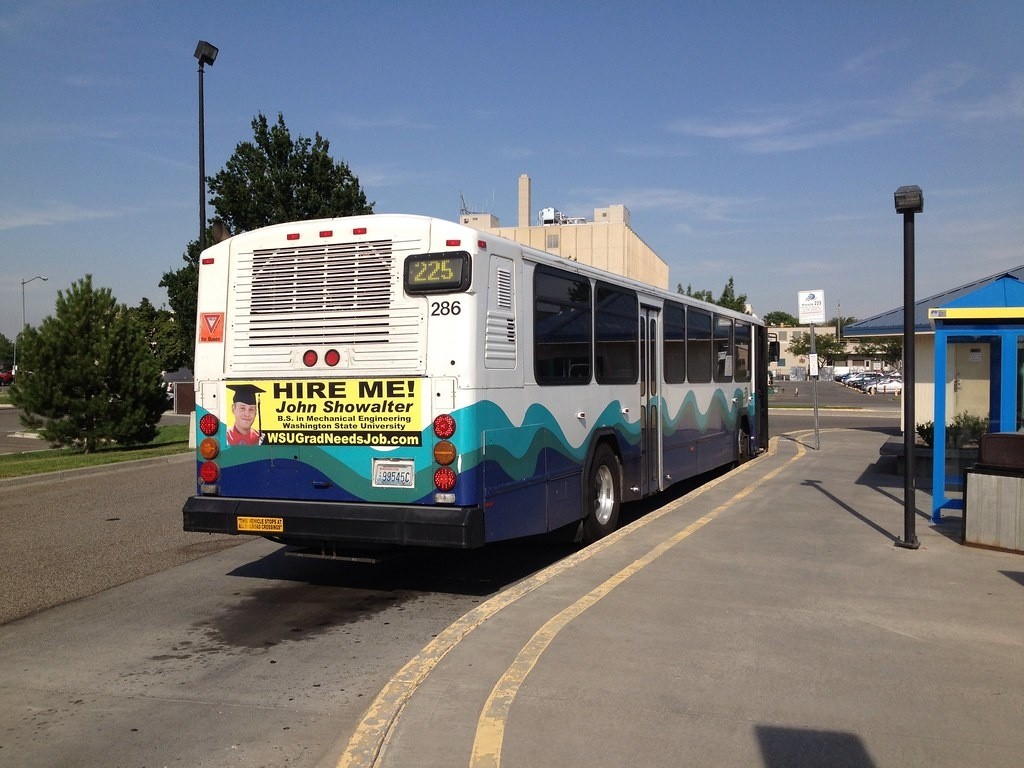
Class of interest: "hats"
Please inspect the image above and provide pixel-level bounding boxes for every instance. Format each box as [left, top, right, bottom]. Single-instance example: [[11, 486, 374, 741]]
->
[[226, 384, 266, 437]]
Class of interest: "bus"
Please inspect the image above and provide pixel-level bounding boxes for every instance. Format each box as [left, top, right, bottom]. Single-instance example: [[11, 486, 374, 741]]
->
[[181, 213, 780, 564]]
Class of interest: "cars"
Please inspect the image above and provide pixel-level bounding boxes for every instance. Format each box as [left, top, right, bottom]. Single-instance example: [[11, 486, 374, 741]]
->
[[768, 371, 773, 384], [0, 370, 14, 386], [834, 371, 903, 393], [158, 382, 174, 409]]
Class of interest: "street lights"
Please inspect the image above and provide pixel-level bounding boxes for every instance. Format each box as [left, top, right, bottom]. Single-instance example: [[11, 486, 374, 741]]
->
[[894, 184, 925, 551], [21, 276, 48, 374], [193, 40, 220, 251]]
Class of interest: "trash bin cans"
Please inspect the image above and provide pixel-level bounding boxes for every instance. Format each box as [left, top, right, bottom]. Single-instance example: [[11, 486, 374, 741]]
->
[[768, 371, 773, 384]]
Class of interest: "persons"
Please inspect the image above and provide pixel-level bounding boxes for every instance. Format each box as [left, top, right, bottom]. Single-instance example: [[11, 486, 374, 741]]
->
[[226, 384, 267, 445]]
